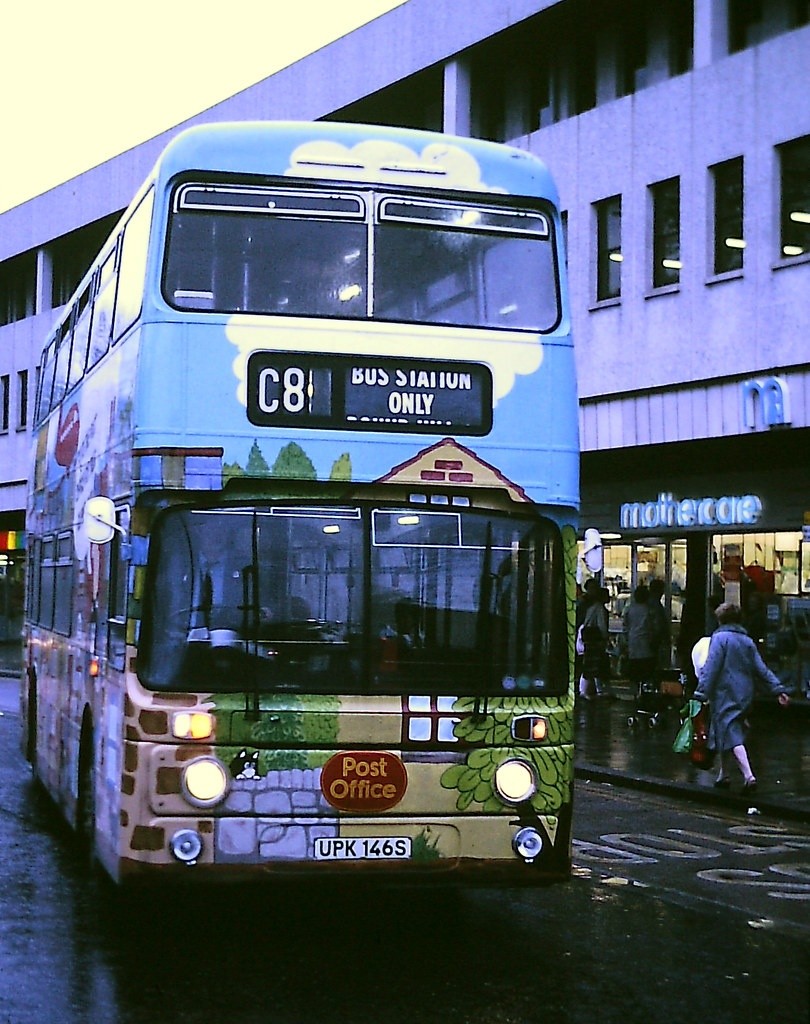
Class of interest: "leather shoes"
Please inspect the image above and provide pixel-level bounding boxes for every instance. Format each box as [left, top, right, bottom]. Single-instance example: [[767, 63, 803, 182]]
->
[[740, 777, 758, 796], [714, 777, 730, 787]]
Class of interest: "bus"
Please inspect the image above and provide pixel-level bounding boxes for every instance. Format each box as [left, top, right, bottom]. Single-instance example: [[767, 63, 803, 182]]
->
[[18, 120, 612, 882]]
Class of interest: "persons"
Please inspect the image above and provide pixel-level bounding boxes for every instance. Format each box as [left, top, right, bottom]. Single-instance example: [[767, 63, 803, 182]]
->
[[775, 615, 800, 688], [169, 518, 273, 630], [576, 575, 707, 706], [742, 576, 767, 643], [691, 602, 790, 790]]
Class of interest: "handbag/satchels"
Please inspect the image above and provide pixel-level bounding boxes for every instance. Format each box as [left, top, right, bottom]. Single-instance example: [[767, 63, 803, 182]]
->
[[673, 700, 714, 770], [581, 626, 611, 679]]
